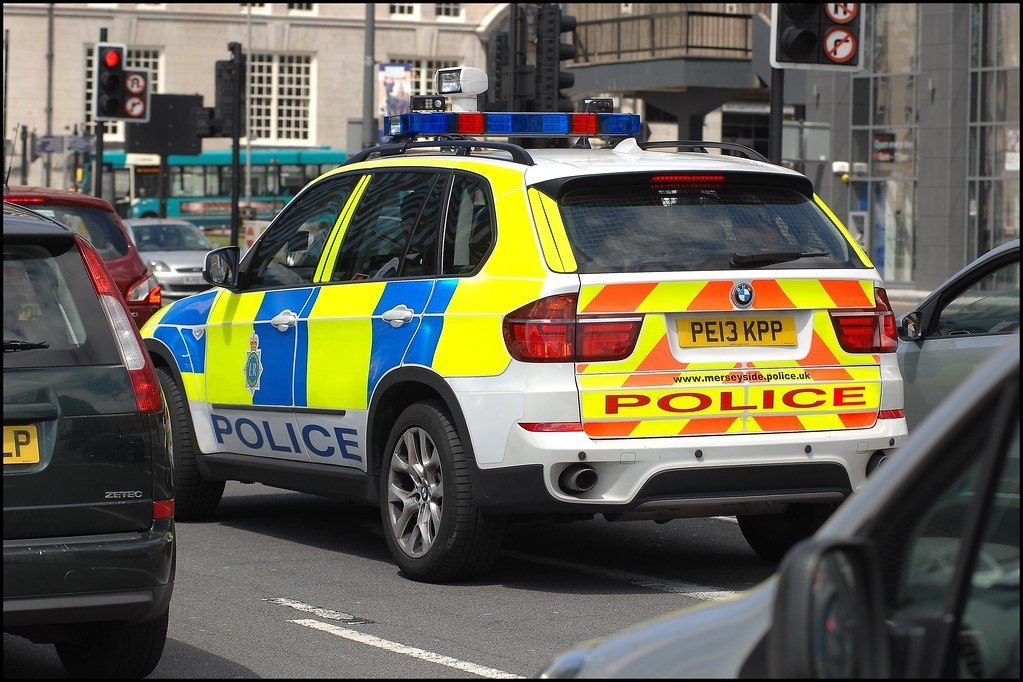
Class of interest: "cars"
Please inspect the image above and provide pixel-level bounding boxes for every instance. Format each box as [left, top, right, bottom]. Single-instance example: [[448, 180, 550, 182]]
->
[[122, 218, 218, 299], [527, 340, 1022, 681], [892, 239, 1023, 515]]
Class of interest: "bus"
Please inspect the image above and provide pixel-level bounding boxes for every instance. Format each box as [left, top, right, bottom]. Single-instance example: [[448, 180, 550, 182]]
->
[[103, 151, 347, 227]]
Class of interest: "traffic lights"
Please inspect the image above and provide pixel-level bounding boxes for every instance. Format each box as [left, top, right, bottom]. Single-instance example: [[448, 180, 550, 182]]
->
[[770, 3, 820, 69], [94, 43, 126, 121], [487, 30, 511, 107], [539, 8, 577, 110], [215, 59, 247, 137]]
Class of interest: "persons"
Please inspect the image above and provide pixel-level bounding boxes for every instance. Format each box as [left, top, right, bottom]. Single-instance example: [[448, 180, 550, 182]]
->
[[211, 264, 222, 281], [164, 232, 177, 246], [401, 186, 438, 252], [134, 231, 143, 249]]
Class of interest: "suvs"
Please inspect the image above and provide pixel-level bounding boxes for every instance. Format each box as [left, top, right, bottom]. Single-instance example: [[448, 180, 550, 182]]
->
[[4, 184, 163, 331], [3, 204, 177, 679], [141, 65, 910, 580]]
